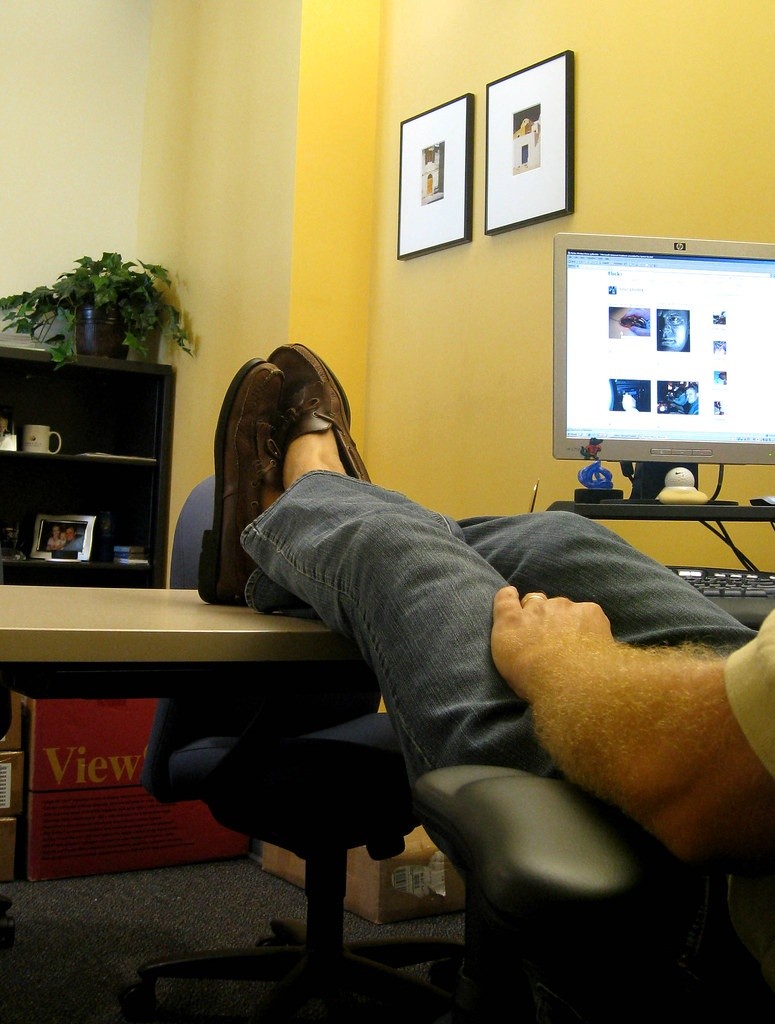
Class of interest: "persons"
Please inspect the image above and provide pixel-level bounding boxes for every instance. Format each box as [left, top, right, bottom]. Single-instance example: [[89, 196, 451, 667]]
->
[[200, 345, 775, 995], [686, 386, 699, 415], [46, 524, 83, 551], [657, 311, 688, 351]]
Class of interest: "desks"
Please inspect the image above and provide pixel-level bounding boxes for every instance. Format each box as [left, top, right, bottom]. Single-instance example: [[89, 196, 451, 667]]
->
[[0, 585, 362, 701]]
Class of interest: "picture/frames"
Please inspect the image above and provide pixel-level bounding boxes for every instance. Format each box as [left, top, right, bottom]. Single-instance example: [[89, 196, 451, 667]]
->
[[483, 50, 574, 236], [30, 514, 97, 561], [397, 93, 473, 261]]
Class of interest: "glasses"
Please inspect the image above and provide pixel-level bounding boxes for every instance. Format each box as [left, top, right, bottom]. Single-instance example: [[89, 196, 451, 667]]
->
[[657, 315, 688, 326]]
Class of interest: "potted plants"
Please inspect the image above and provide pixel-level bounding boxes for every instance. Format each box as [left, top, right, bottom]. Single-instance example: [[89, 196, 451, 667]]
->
[[0, 252, 193, 372]]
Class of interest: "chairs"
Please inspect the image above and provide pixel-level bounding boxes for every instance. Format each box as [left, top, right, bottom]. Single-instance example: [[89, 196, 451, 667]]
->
[[117, 478, 479, 1024], [414, 762, 775, 1024]]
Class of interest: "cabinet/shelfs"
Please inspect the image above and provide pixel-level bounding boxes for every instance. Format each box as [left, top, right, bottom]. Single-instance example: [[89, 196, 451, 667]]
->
[[0, 346, 177, 589]]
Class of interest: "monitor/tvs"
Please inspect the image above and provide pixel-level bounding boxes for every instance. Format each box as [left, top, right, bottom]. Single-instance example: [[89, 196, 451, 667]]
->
[[552, 232, 774, 503]]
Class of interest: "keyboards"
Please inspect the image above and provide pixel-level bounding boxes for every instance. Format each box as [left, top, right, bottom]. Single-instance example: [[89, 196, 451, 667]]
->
[[666, 564, 774, 631]]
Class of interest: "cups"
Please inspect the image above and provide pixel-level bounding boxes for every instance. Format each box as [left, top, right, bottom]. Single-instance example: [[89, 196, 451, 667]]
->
[[22, 424, 62, 454], [98, 510, 118, 539], [0, 520, 19, 562]]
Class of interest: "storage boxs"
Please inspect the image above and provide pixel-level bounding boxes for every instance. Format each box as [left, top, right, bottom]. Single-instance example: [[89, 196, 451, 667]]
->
[[0, 751, 24, 816], [18, 695, 159, 792], [0, 817, 16, 882], [0, 691, 22, 749], [262, 825, 465, 926], [15, 790, 250, 882]]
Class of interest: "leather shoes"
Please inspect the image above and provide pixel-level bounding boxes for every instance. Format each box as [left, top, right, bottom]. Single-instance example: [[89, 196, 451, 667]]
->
[[197, 358, 286, 605], [267, 342, 372, 486]]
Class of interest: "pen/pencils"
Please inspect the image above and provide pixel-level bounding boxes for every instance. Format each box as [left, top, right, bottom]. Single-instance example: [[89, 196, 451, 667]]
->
[[527, 478, 540, 513]]
